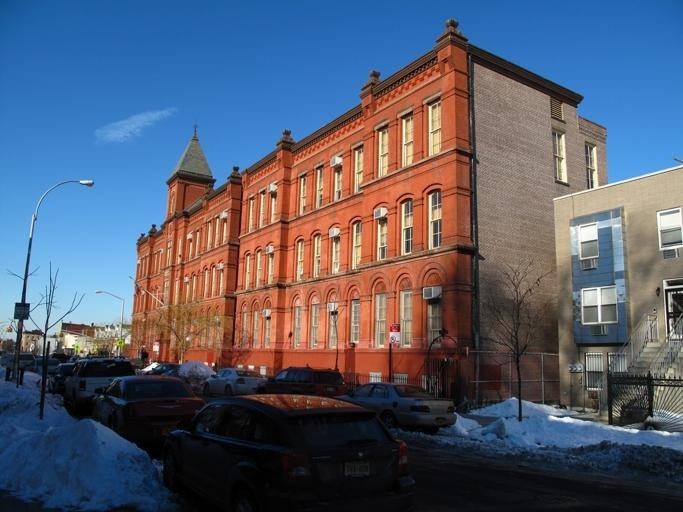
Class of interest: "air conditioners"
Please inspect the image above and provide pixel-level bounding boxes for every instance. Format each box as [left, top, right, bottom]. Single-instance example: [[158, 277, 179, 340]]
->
[[422, 288, 444, 300], [389, 333, 400, 343], [328, 228, 340, 237], [220, 211, 226, 220], [330, 157, 340, 166], [266, 246, 273, 253], [216, 262, 223, 269], [328, 303, 339, 313], [374, 208, 385, 219], [266, 184, 276, 191]]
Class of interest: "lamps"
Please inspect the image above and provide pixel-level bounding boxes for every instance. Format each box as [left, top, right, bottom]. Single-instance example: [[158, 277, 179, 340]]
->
[[262, 309, 270, 316]]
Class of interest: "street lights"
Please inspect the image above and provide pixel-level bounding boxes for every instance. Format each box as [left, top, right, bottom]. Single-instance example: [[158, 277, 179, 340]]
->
[[11, 179, 95, 383], [96, 290, 125, 357]]
